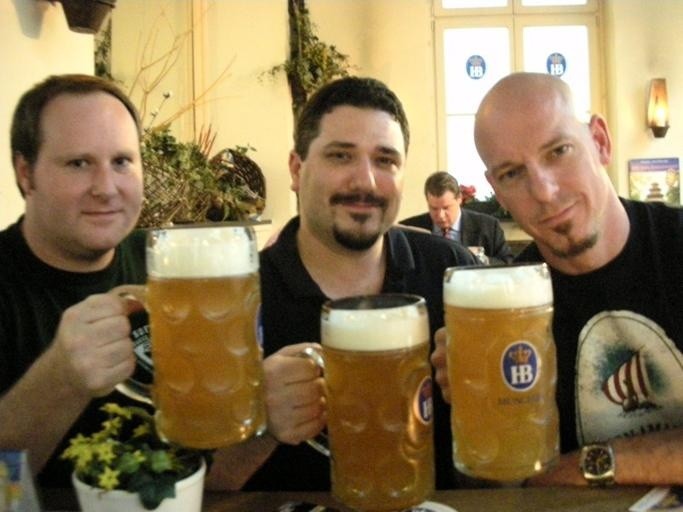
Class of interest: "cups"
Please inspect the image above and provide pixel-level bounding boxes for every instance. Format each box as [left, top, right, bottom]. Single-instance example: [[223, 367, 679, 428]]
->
[[440, 258, 561, 480], [292, 288, 434, 510], [104, 222, 268, 451]]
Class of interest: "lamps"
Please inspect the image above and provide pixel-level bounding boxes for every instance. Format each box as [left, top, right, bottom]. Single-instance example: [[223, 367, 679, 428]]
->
[[647, 77, 671, 142]]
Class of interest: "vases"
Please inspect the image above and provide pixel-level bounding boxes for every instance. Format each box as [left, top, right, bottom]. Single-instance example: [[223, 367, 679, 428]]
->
[[70, 460, 208, 511]]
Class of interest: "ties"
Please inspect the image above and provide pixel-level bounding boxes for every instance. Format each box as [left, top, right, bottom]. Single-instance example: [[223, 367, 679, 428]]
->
[[441, 227, 452, 240]]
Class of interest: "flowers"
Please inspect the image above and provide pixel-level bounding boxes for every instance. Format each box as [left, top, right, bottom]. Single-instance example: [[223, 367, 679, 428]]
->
[[63, 403, 203, 510]]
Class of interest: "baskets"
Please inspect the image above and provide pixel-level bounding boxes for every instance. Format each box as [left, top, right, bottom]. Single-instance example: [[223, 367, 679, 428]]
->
[[137, 149, 267, 228]]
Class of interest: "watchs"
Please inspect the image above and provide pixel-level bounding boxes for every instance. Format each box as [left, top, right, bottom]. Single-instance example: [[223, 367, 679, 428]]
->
[[256, 426, 281, 451], [579, 441, 615, 490]]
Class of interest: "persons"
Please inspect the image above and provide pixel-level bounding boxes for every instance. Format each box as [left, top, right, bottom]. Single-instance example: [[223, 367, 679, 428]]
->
[[0, 75, 147, 487], [432, 73, 683, 490], [399, 172, 514, 266], [204, 76, 486, 492]]
